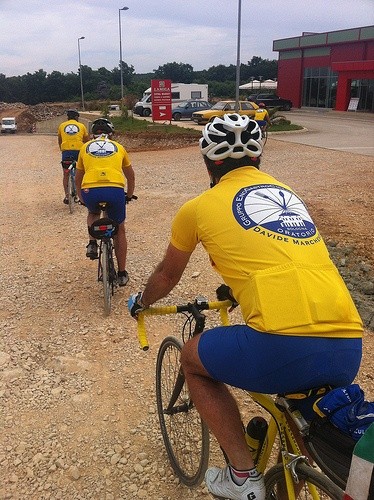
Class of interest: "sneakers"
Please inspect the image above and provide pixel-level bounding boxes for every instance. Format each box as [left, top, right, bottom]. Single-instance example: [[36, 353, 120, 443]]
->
[[204, 466, 266, 500]]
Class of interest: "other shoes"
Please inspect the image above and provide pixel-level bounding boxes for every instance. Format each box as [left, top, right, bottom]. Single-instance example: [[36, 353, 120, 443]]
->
[[117, 271, 129, 285], [63, 198, 69, 204], [86, 243, 98, 257]]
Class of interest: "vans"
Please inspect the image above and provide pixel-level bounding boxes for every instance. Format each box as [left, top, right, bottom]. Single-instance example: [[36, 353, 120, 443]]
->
[[0, 117, 17, 133]]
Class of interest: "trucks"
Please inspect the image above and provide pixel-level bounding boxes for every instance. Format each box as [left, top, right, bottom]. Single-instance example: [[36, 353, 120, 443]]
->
[[134, 83, 209, 116]]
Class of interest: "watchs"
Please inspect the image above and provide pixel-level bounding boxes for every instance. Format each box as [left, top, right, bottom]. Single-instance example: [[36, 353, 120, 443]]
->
[[136, 293, 148, 309]]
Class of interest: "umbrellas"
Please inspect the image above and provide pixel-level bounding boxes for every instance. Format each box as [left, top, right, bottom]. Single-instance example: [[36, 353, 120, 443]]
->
[[239, 79, 277, 93]]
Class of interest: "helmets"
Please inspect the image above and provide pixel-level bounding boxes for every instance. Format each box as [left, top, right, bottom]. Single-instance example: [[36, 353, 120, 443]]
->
[[259, 103, 265, 108], [198, 113, 264, 161], [91, 117, 115, 135], [67, 109, 79, 118]]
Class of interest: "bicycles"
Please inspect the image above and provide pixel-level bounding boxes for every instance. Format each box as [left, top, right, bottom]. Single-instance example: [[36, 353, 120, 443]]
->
[[258, 120, 273, 146], [62, 157, 79, 214], [72, 192, 138, 315], [135, 284, 374, 500]]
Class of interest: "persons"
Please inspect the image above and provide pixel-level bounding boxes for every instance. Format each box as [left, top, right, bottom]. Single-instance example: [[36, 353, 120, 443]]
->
[[128, 114, 365, 500], [254, 103, 272, 132], [75, 118, 135, 286], [57, 110, 90, 204]]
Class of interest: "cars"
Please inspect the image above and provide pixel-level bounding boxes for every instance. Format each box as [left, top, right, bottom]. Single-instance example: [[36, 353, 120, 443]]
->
[[247, 94, 292, 111], [172, 100, 212, 121], [191, 100, 259, 125]]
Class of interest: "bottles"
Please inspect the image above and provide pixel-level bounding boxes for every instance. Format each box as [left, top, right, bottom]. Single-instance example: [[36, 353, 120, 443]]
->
[[245, 416, 268, 466]]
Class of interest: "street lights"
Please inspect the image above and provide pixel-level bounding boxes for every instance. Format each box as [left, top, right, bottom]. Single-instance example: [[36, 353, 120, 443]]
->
[[77, 37, 85, 108], [251, 76, 263, 94], [119, 7, 129, 99]]
